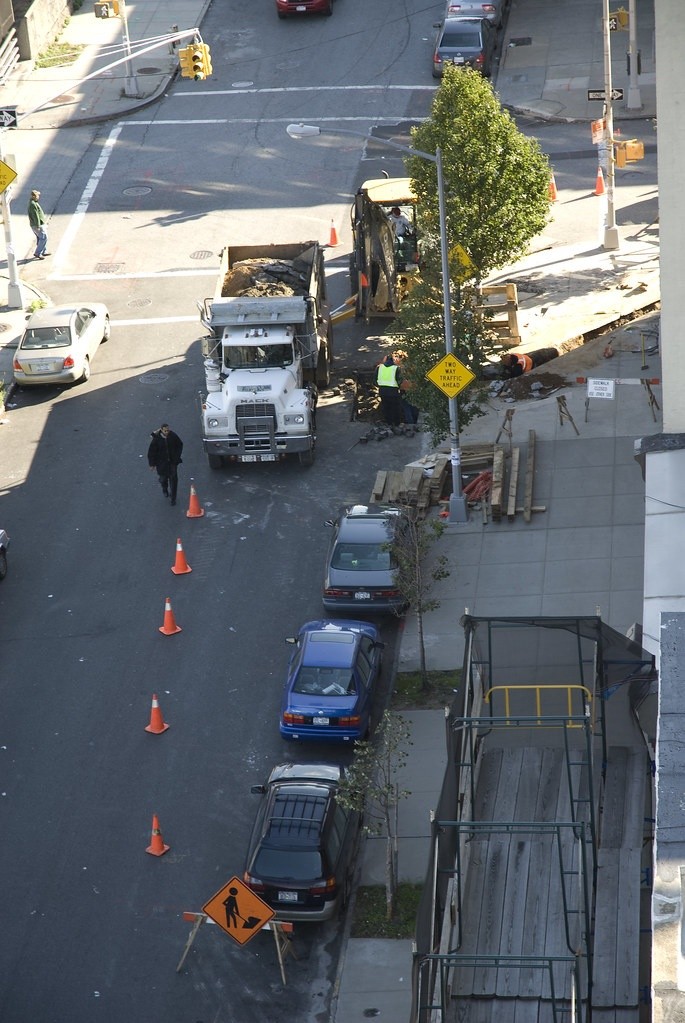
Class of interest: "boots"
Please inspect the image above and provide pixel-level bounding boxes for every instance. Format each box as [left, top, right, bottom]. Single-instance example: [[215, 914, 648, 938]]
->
[[170, 485, 179, 505], [159, 479, 170, 497]]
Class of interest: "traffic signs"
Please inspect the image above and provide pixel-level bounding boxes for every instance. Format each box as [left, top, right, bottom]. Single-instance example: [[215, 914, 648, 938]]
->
[[586, 87, 623, 101], [0, 107, 18, 129]]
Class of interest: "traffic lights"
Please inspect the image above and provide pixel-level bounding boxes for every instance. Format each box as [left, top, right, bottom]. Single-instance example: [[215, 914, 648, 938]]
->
[[179, 46, 195, 79], [192, 46, 207, 82], [601, 16, 619, 33], [94, 3, 109, 18]]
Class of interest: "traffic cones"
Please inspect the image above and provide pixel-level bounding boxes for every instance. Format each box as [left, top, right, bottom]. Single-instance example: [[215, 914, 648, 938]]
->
[[548, 172, 559, 203], [326, 218, 344, 246], [170, 537, 192, 575], [592, 166, 607, 196], [145, 814, 170, 857], [186, 485, 204, 517], [158, 597, 183, 636], [144, 694, 170, 734]]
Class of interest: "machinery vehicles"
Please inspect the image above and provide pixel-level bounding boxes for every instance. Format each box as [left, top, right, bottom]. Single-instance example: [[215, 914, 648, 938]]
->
[[329, 169, 466, 327]]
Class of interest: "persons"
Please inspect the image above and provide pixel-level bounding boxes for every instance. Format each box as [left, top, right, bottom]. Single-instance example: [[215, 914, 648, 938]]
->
[[500, 354, 537, 377], [148, 424, 183, 506], [374, 355, 419, 424], [28, 190, 51, 260], [385, 208, 411, 236]]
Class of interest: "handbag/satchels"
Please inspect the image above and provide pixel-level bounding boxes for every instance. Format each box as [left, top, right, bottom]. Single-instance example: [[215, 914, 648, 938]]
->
[[400, 378, 415, 391]]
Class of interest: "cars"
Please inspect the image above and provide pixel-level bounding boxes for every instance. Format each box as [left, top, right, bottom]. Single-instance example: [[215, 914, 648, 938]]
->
[[446, 0, 504, 31], [242, 759, 364, 922], [432, 16, 499, 79], [13, 301, 110, 390], [323, 502, 411, 618], [278, 618, 388, 746], [276, 0, 333, 19]]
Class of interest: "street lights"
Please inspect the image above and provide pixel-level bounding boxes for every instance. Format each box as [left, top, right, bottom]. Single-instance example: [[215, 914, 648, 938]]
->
[[286, 122, 470, 522]]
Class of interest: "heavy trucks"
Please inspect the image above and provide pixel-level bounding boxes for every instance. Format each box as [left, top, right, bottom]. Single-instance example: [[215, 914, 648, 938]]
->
[[197, 239, 333, 472]]
